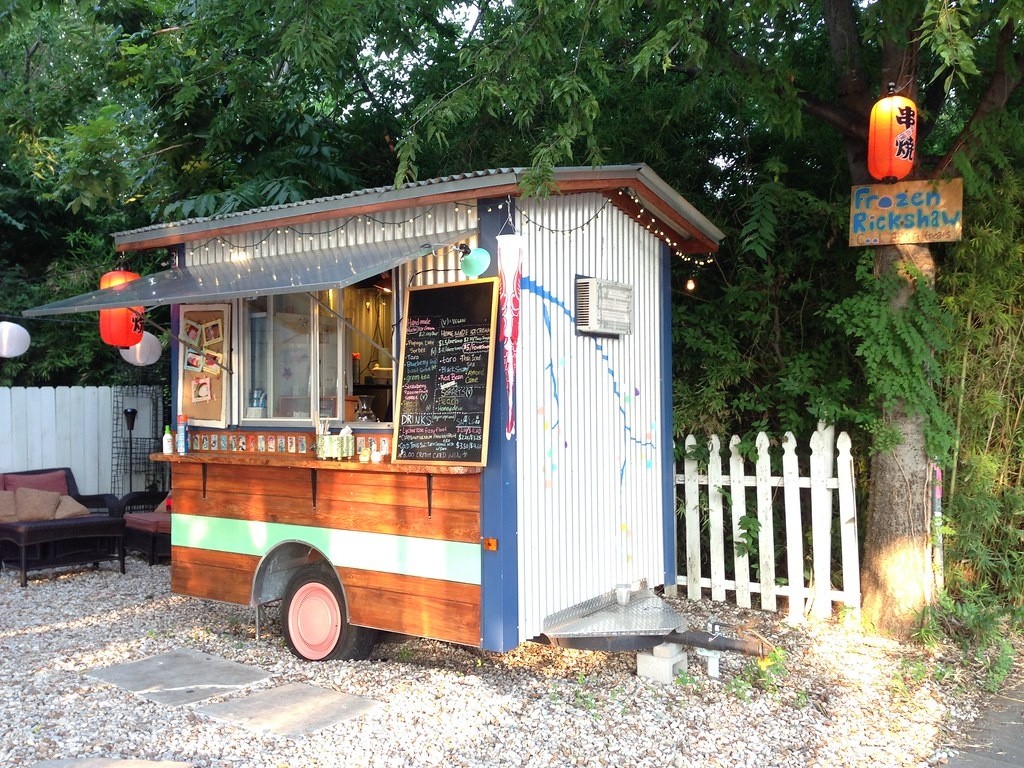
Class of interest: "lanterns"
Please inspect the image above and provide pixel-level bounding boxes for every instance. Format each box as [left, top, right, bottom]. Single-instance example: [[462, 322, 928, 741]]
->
[[0, 320, 31, 358], [868, 96, 917, 185], [119, 330, 162, 366], [100, 271, 145, 350]]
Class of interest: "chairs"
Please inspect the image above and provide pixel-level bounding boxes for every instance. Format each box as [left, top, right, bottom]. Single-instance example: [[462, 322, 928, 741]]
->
[[119, 489, 172, 567]]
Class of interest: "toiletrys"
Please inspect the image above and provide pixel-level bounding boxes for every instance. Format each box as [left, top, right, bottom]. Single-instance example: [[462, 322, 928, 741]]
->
[[162, 425, 175, 457]]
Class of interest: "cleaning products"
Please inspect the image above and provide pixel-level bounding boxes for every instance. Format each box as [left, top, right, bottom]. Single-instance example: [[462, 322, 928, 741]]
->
[[176, 413, 190, 456]]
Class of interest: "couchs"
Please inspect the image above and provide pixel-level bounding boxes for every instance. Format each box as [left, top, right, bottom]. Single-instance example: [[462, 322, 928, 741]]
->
[[0, 466, 128, 572]]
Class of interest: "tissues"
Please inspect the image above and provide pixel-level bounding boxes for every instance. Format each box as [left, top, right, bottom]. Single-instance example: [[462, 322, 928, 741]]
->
[[316, 425, 356, 462]]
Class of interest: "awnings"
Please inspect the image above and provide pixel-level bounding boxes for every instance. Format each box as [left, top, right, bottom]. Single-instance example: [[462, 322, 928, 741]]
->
[[22, 227, 481, 317]]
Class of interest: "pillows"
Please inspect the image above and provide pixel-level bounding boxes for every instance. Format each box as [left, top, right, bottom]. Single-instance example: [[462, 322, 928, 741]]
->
[[53, 495, 90, 520], [0, 490, 18, 523], [3, 469, 69, 498], [14, 486, 61, 523]]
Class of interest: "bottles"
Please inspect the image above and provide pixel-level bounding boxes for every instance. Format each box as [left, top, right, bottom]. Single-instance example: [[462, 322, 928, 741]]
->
[[353, 394, 376, 422]]
